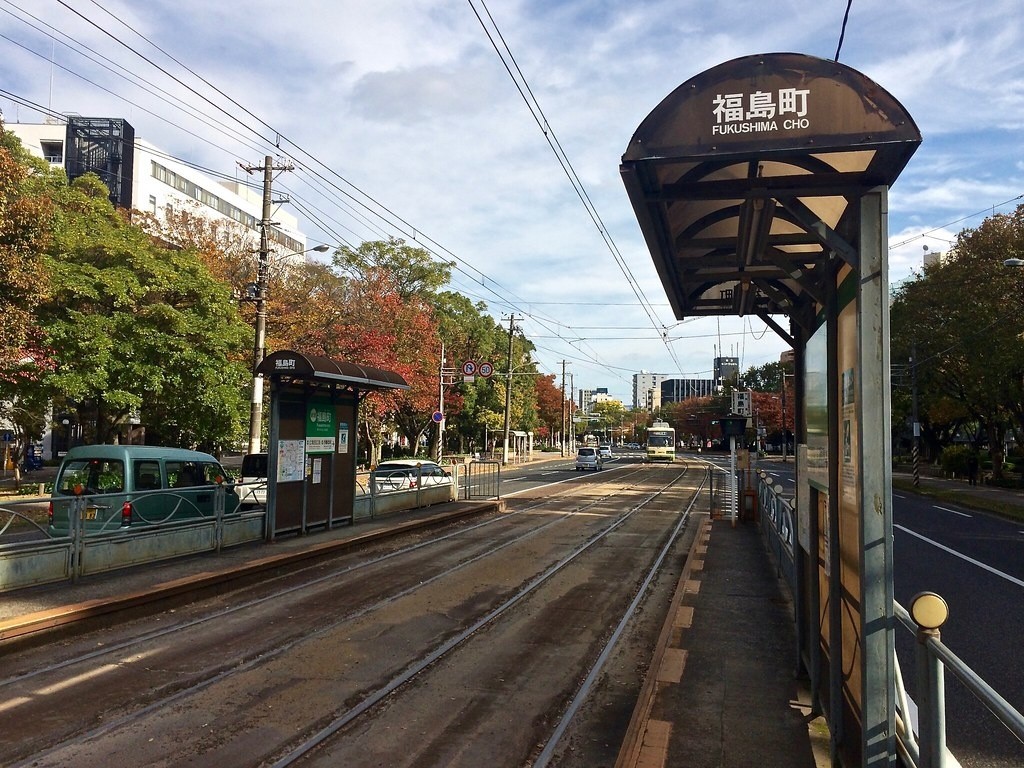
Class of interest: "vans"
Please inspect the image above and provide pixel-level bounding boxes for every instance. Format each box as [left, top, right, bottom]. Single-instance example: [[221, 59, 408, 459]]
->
[[237, 451, 268, 508], [575, 447, 602, 472], [46, 445, 241, 541]]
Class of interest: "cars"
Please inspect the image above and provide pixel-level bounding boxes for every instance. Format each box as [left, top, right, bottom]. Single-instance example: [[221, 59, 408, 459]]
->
[[599, 444, 612, 459], [365, 460, 455, 500], [612, 442, 648, 450]]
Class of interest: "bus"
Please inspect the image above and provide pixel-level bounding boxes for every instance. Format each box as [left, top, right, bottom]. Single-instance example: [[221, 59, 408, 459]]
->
[[646, 421, 675, 464]]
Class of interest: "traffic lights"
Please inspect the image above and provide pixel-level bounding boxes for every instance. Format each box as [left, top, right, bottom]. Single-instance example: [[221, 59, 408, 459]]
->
[[724, 419, 742, 436]]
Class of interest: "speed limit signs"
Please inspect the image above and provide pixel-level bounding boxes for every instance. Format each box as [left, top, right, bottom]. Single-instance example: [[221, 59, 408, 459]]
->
[[479, 363, 494, 378]]
